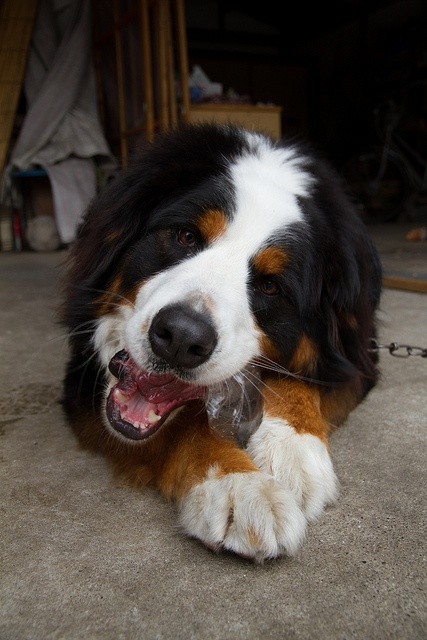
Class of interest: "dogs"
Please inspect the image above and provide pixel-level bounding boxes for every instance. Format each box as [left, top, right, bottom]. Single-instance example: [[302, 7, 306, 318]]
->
[[39, 114, 385, 568]]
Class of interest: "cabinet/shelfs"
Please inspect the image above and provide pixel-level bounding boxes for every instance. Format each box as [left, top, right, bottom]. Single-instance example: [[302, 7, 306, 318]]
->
[[182, 102, 280, 146]]
